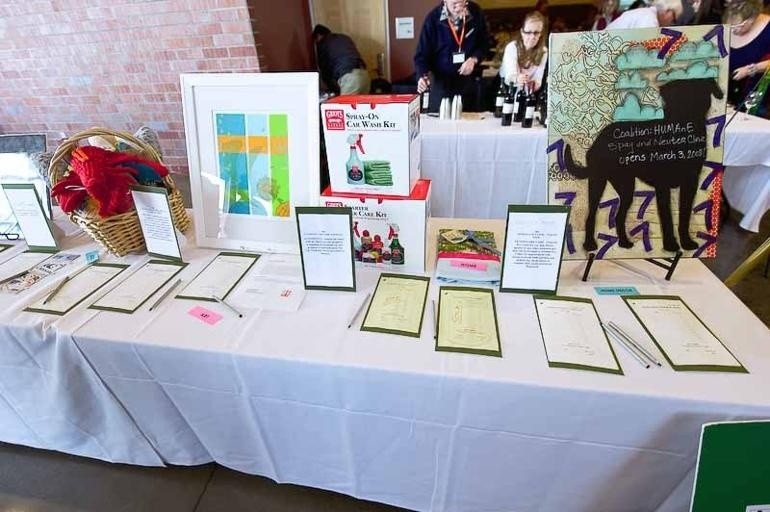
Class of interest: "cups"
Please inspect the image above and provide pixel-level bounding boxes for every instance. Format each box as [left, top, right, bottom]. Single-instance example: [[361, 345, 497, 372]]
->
[[439, 94, 464, 121]]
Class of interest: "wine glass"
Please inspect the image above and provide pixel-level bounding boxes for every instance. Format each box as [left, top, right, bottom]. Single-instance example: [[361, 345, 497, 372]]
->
[[742, 89, 764, 121]]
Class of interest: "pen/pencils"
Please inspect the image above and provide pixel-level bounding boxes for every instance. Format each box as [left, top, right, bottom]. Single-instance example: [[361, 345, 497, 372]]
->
[[608, 321, 662, 367], [347, 294, 371, 328], [600, 322, 650, 368], [212, 294, 243, 317], [149, 279, 182, 311], [42, 276, 70, 304], [432, 300, 438, 339]]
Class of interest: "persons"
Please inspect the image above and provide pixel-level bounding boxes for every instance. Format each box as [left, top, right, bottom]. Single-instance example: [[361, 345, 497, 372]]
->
[[413, 0, 490, 113], [722, 0, 769, 118], [592, 0, 623, 31], [312, 24, 371, 96], [599, 0, 683, 32], [628, 0, 646, 10], [492, 10, 548, 110]]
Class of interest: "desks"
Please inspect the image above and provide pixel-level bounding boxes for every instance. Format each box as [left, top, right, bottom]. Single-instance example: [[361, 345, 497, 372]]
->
[[0, 205, 770, 511], [420, 110, 548, 220], [723, 110, 770, 234]]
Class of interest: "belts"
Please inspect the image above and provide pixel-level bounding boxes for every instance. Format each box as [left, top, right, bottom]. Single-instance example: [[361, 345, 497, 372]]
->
[[352, 65, 368, 70]]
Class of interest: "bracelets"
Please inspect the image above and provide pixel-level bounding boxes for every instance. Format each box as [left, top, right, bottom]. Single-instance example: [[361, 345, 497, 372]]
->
[[747, 64, 757, 77]]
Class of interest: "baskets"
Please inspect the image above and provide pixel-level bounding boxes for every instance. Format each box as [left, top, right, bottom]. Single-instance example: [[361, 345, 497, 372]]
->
[[47, 127, 191, 257]]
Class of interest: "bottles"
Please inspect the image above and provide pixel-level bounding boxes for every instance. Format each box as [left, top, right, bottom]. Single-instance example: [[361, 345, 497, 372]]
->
[[360, 230, 384, 263], [495, 76, 548, 128], [419, 73, 430, 114]]
